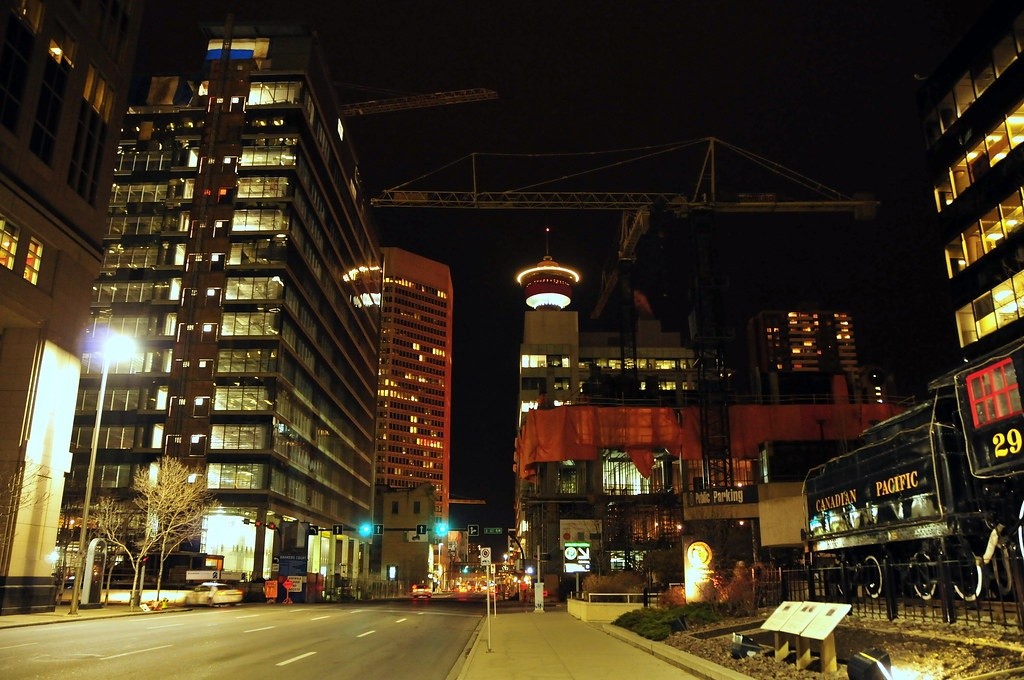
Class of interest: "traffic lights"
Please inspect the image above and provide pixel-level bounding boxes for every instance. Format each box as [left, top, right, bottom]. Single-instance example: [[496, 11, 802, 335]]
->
[[268, 522, 276, 529], [256, 519, 262, 526]]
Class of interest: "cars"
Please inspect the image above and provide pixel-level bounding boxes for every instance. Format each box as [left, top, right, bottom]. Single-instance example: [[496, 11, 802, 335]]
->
[[183, 582, 243, 608], [411, 583, 433, 600]]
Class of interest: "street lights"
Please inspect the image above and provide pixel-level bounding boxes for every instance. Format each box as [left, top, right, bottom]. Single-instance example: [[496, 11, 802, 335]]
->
[[67, 333, 139, 617]]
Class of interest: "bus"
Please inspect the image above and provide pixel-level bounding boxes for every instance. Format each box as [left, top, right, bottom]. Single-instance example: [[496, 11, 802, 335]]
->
[[101, 550, 224, 590], [797, 336, 1024, 604]]
